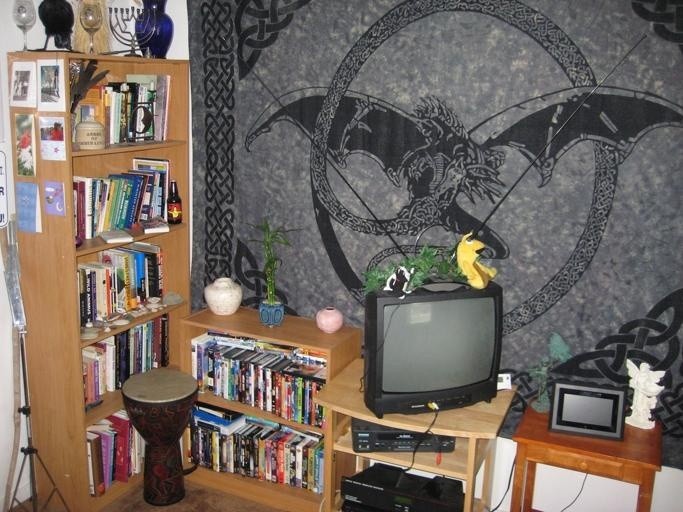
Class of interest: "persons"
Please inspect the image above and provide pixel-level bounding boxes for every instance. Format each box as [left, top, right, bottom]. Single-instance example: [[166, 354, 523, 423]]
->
[[626, 357, 665, 421]]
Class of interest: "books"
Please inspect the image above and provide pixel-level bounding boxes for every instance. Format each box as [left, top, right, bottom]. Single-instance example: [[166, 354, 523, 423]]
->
[[82, 312, 169, 405], [188, 327, 324, 430], [72, 158, 171, 244], [76, 242, 164, 327], [74, 72, 171, 146], [190, 401, 325, 495], [85, 408, 146, 497]]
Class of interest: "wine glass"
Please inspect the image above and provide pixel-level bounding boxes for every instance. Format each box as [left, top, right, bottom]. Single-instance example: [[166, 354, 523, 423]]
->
[[11, 0, 37, 52], [79, 4, 102, 54]]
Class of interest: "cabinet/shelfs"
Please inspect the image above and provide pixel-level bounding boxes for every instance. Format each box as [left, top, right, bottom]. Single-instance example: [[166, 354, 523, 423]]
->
[[7, 51, 189, 511]]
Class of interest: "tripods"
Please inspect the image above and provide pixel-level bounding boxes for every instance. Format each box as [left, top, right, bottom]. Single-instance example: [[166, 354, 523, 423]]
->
[[9, 406, 71, 512]]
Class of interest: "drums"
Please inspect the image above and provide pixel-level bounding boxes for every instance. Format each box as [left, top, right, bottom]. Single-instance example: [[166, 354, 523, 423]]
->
[[122, 368, 198, 505]]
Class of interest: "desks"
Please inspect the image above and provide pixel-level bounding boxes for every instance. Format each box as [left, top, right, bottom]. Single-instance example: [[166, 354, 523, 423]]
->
[[511, 405, 662, 512]]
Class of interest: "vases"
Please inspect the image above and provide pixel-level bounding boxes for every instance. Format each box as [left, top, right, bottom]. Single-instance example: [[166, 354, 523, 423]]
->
[[203, 278, 243, 316], [316, 306, 344, 334]]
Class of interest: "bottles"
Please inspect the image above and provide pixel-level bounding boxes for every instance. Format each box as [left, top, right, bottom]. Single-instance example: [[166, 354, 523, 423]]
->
[[166, 180, 183, 225], [74, 104, 107, 150]]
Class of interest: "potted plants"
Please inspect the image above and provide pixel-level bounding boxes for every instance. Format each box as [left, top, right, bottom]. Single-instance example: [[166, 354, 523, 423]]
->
[[245, 216, 305, 327]]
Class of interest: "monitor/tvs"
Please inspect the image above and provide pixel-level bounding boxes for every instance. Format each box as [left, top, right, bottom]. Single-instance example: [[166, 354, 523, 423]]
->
[[364, 280, 504, 418]]
